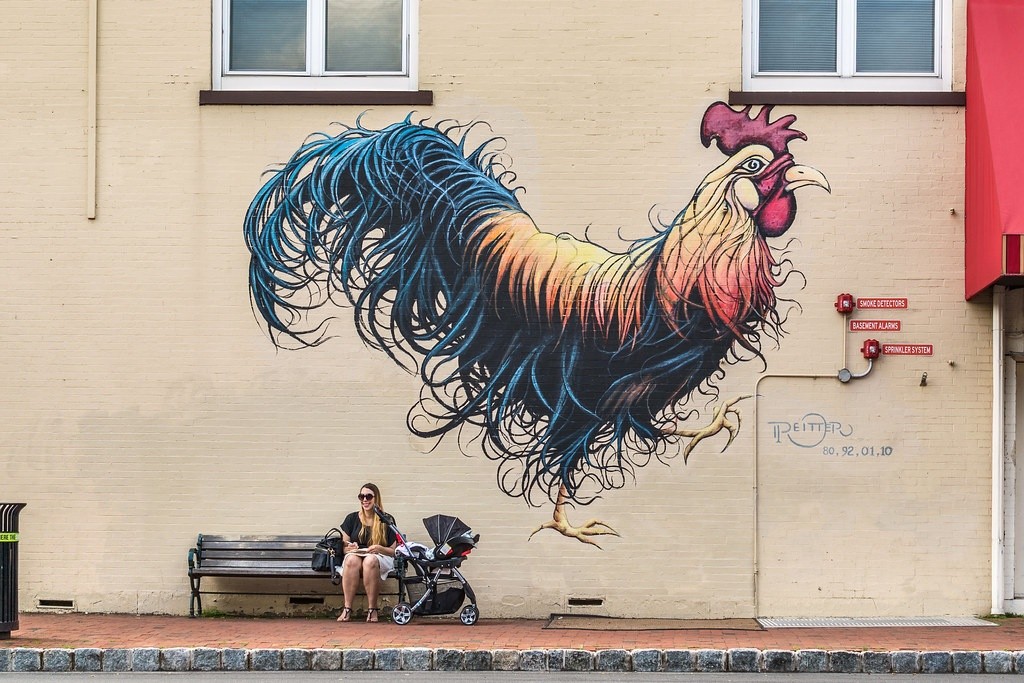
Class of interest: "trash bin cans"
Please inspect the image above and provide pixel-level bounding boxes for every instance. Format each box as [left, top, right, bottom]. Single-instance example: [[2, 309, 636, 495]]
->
[[1, 503, 29, 639]]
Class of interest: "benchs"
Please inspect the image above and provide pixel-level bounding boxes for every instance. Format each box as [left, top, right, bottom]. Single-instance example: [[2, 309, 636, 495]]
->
[[187, 533, 408, 620]]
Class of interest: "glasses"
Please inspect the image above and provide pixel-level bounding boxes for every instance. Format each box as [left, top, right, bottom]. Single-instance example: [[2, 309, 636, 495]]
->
[[358, 493, 375, 501]]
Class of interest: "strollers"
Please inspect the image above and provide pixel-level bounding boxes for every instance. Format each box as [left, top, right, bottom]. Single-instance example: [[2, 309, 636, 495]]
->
[[372, 505, 480, 625]]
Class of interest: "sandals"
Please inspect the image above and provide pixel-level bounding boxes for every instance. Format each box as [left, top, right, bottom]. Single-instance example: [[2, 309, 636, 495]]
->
[[366, 608, 380, 623], [336, 607, 353, 622]]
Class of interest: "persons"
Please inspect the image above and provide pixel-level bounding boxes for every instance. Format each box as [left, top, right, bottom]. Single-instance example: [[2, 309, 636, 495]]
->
[[337, 483, 397, 623]]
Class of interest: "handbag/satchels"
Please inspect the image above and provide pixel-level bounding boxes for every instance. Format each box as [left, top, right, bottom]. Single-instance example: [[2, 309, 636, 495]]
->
[[312, 527, 345, 572]]
